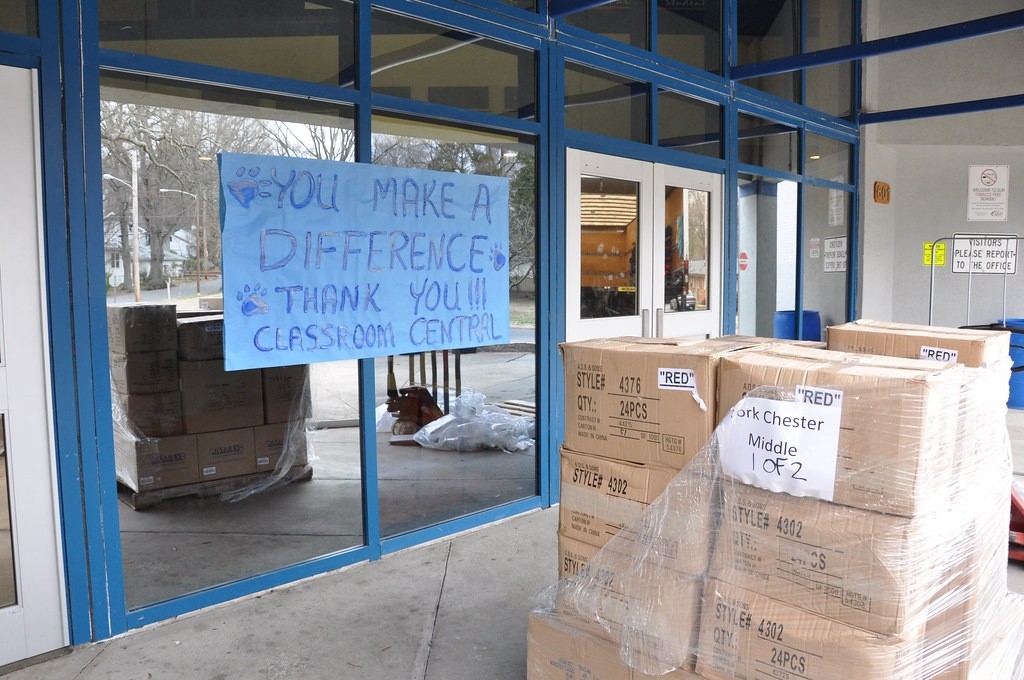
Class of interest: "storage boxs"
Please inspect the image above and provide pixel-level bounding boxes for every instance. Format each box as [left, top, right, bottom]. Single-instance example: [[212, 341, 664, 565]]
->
[[525, 318, 1023, 680], [106, 294, 312, 494]]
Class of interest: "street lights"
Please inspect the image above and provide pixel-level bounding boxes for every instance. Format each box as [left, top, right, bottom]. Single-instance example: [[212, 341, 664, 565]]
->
[[103, 173, 141, 302], [158, 188, 204, 296]]
